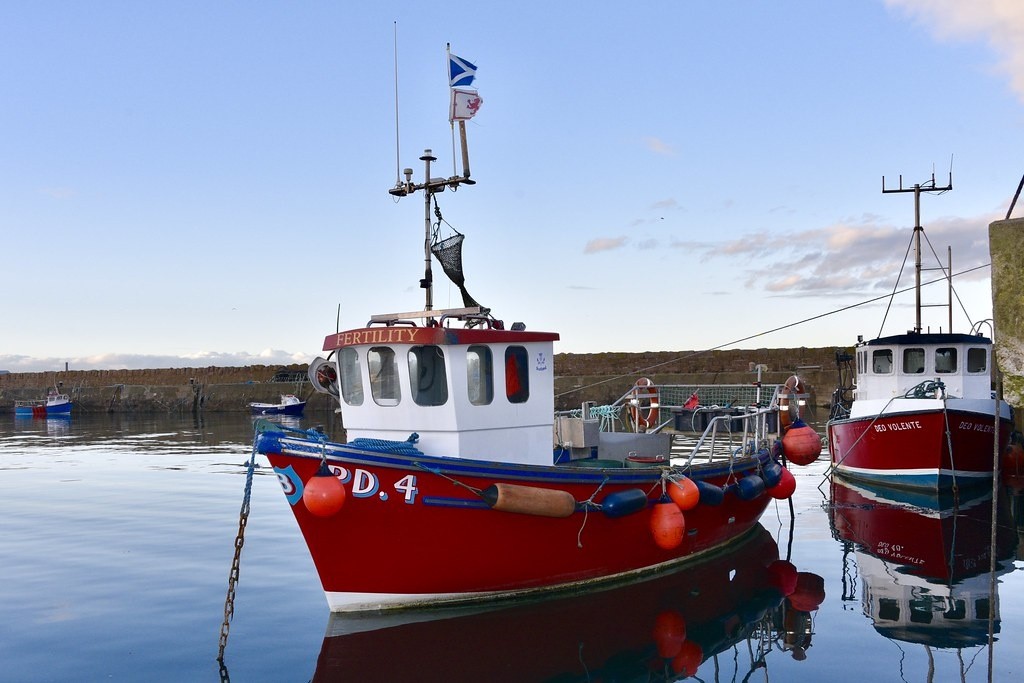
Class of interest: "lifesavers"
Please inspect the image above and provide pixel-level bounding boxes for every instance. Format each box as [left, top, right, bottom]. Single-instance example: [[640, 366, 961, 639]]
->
[[629, 378, 660, 426], [780, 376, 806, 430]]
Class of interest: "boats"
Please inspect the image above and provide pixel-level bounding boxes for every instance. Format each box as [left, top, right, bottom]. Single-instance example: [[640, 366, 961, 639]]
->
[[825, 474, 1024, 683], [249, 395, 306, 415], [827, 152, 1012, 494], [257, 21, 823, 615], [311, 521, 825, 683], [15, 380, 73, 415]]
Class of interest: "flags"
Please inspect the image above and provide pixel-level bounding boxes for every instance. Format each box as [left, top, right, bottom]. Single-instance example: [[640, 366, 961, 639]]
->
[[448, 51, 481, 120]]
